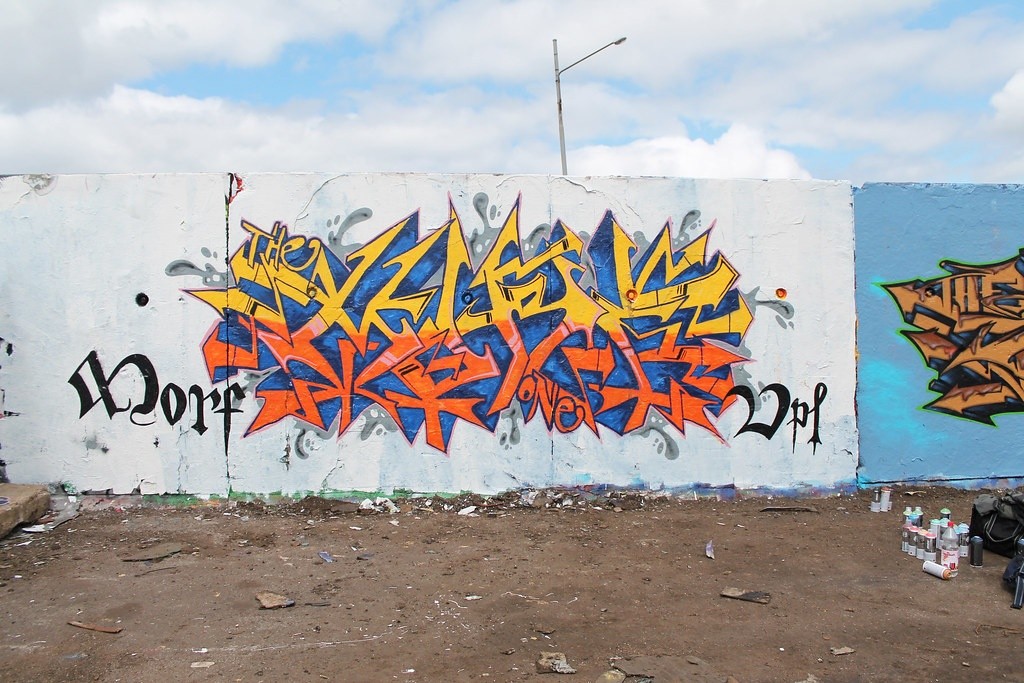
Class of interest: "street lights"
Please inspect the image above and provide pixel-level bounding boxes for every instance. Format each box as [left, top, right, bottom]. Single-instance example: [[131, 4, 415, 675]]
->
[[555, 36, 627, 175]]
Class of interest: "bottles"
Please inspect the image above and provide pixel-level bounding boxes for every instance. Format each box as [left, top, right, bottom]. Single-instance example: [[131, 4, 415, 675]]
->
[[970, 536, 984, 568], [941, 522, 959, 578], [902, 506, 969, 563], [922, 560, 952, 579], [871, 488, 892, 512]]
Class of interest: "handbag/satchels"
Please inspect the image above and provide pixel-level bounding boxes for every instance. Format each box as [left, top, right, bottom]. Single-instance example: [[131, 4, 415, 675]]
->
[[969, 491, 1024, 559], [1003, 538, 1024, 608]]
